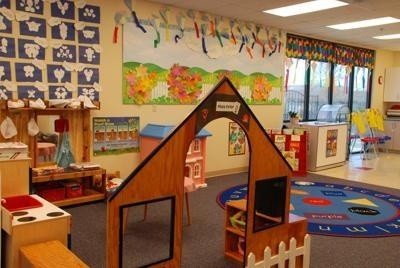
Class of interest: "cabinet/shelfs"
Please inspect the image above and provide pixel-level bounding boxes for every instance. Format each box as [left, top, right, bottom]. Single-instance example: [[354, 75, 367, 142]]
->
[[32, 166, 106, 207], [223, 199, 248, 262]]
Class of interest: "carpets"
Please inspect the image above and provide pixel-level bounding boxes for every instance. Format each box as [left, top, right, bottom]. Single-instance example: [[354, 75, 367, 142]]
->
[[216, 178, 399, 237]]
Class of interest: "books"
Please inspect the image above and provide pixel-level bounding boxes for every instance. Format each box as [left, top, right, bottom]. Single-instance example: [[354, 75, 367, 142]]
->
[[32, 164, 64, 177], [1, 195, 43, 213], [70, 161, 100, 171]]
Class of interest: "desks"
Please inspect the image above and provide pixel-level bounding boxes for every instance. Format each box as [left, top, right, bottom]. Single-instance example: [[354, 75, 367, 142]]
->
[[107, 176, 193, 234], [300, 120, 348, 172]]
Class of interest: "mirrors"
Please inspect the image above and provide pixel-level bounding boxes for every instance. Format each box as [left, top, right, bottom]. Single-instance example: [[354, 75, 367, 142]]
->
[[35, 113, 60, 165]]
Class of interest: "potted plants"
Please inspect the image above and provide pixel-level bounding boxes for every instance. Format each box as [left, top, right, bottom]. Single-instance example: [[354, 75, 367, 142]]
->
[[288, 112, 299, 127]]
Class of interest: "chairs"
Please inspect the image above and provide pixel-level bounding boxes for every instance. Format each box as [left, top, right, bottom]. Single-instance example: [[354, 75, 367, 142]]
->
[[351, 108, 392, 167]]
